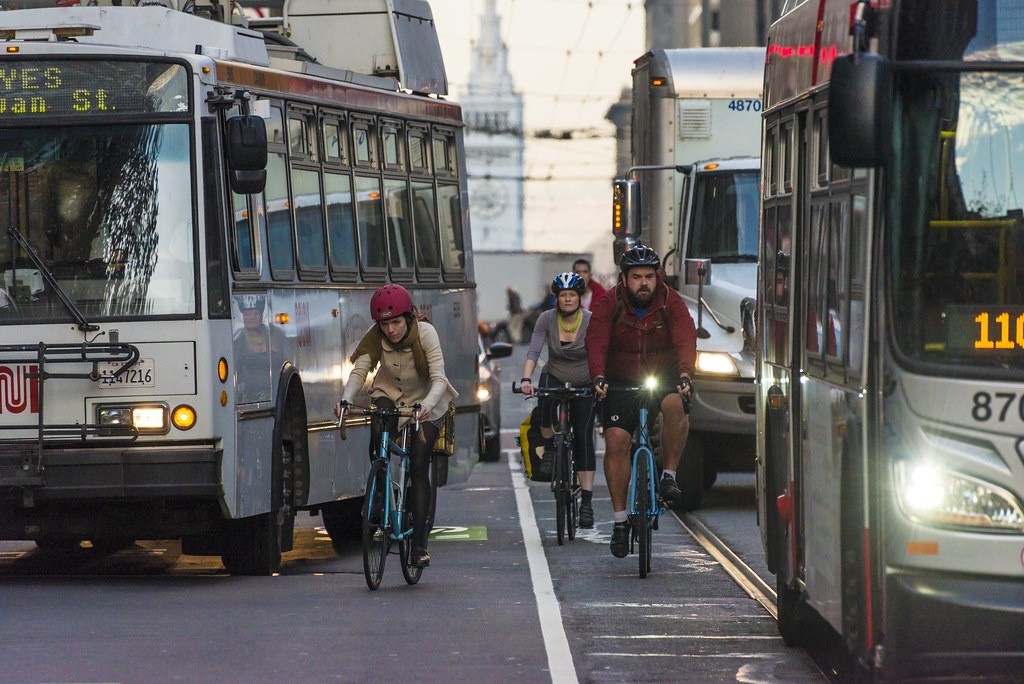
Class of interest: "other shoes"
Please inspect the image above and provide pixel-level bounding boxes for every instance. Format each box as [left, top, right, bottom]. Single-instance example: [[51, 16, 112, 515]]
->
[[372, 527, 383, 541], [412, 546, 430, 563]]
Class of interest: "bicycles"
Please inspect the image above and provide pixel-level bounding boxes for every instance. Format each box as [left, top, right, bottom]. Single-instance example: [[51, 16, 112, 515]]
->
[[512, 377, 600, 547], [331, 394, 456, 591], [583, 380, 694, 581]]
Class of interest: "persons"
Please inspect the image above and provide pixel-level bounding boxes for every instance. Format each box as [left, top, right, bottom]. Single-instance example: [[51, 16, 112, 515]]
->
[[332, 282, 459, 567], [520, 270, 596, 527], [573, 259, 606, 315], [584, 245, 696, 558]]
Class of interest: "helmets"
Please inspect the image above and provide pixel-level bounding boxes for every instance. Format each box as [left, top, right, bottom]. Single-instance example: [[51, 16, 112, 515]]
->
[[620, 245, 660, 273], [370, 284, 413, 320], [551, 272, 585, 295]]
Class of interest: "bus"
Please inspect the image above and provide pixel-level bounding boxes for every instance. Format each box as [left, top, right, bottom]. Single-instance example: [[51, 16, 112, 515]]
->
[[1, 0, 482, 575], [745, 1, 1024, 684]]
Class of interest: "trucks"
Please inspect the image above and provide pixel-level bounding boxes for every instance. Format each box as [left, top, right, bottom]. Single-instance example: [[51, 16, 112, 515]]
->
[[608, 43, 773, 510]]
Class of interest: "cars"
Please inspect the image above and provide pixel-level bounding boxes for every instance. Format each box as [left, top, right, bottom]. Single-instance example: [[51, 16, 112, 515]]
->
[[475, 323, 514, 461]]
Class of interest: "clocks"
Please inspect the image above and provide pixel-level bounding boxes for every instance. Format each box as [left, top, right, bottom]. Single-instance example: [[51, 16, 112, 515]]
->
[[469, 176, 509, 218]]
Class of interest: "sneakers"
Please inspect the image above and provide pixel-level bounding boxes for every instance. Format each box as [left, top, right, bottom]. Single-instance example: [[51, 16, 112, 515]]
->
[[539, 446, 554, 476], [610, 521, 632, 557], [660, 473, 682, 501], [579, 503, 594, 525]]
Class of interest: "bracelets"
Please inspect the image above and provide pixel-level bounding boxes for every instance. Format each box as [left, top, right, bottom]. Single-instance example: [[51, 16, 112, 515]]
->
[[521, 378, 531, 384]]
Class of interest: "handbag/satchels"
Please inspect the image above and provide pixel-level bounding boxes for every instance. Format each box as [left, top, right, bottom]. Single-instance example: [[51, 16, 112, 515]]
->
[[520, 406, 555, 481]]
